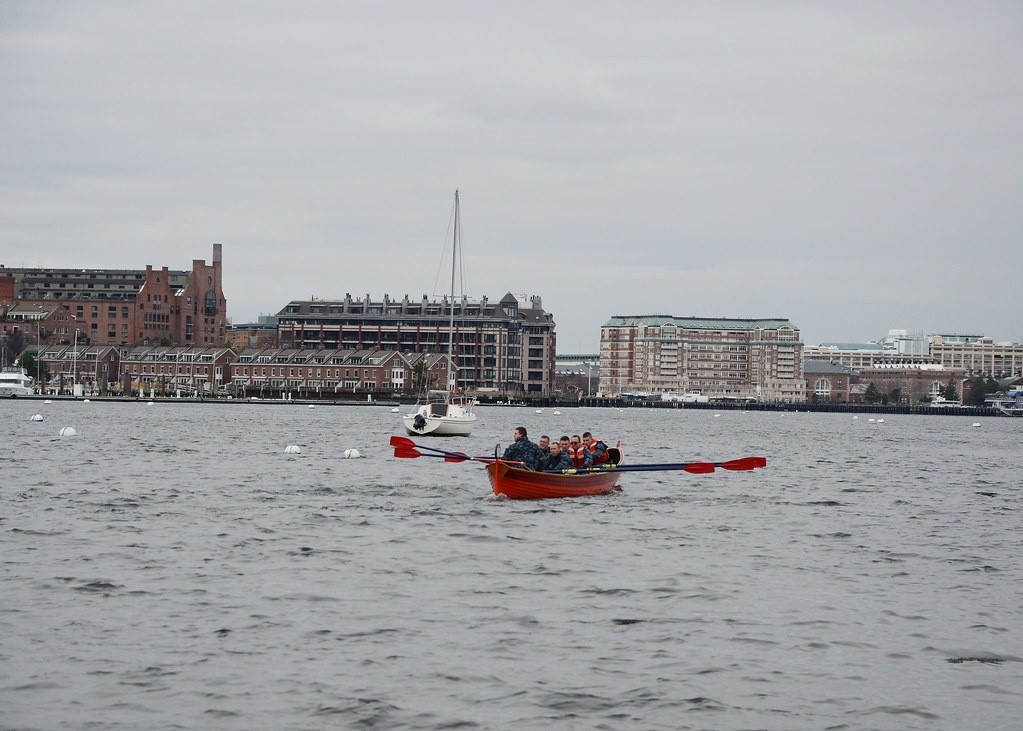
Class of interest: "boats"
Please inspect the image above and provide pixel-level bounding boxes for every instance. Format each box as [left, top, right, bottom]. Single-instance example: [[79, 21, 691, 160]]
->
[[0, 351, 35, 399], [486, 441, 625, 500]]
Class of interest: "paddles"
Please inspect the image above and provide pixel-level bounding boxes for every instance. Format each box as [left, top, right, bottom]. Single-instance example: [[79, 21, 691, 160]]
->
[[543, 457, 766, 477], [390, 434, 525, 466]]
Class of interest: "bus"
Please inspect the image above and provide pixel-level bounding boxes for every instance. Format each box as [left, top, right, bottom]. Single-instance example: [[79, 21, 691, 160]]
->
[[929, 401, 961, 408]]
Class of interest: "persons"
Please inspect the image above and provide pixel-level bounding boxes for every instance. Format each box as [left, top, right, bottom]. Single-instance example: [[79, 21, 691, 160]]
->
[[559, 435, 593, 471], [539, 434, 573, 472], [502, 427, 547, 471], [582, 432, 610, 465]]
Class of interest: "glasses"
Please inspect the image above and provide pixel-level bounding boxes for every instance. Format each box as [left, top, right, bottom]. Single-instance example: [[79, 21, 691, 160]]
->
[[571, 442, 579, 445]]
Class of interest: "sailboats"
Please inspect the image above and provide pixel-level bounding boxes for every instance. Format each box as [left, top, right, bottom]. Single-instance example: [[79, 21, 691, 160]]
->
[[402, 189, 478, 436]]
